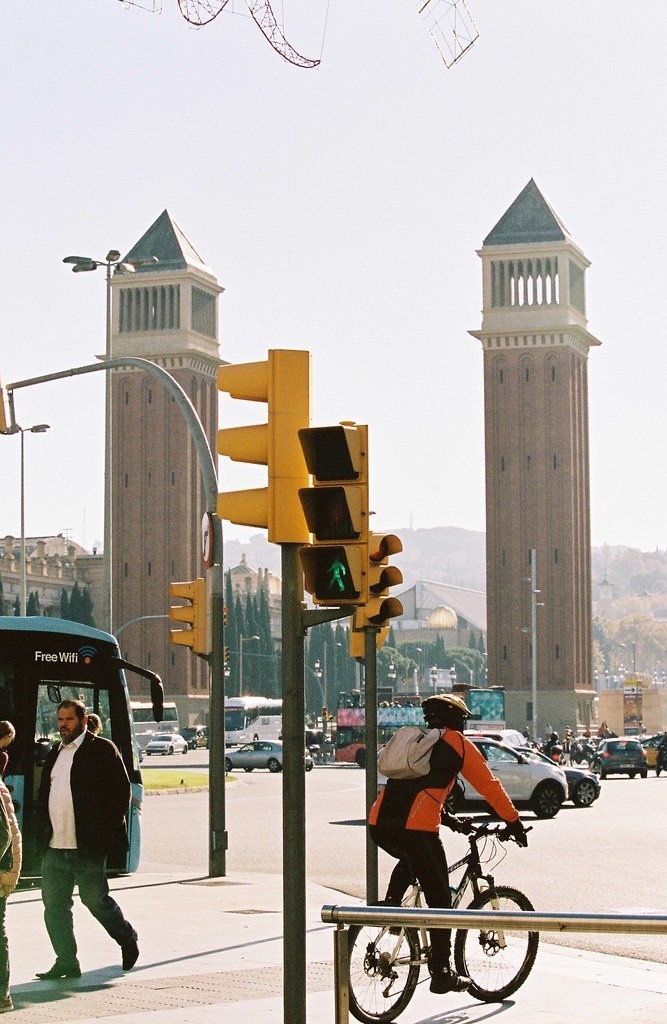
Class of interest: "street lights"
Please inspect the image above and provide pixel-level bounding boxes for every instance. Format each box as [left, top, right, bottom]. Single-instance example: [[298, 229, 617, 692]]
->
[[431, 663, 439, 696], [238, 633, 261, 698], [416, 648, 422, 700], [324, 640, 342, 709], [315, 658, 328, 707], [0, 422, 50, 617], [448, 663, 456, 690], [62, 250, 158, 635], [593, 643, 666, 691], [387, 662, 396, 704]]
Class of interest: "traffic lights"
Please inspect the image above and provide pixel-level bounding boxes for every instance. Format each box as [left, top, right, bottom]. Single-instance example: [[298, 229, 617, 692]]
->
[[215, 350, 313, 547], [296, 422, 371, 606], [223, 608, 228, 627], [347, 535, 406, 661], [168, 577, 215, 655], [328, 713, 333, 721], [222, 645, 229, 662], [322, 706, 328, 722]]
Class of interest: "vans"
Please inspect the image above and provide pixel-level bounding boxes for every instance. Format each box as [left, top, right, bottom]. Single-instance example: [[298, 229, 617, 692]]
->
[[178, 726, 210, 750]]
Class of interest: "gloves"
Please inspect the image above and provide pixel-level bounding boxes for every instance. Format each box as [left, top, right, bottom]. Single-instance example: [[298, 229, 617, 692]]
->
[[500, 819, 529, 848], [441, 810, 474, 833]]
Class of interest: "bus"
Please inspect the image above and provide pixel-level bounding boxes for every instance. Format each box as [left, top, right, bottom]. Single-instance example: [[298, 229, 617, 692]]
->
[[106, 701, 179, 751], [224, 696, 283, 747], [336, 707, 429, 767], [0, 616, 166, 883]]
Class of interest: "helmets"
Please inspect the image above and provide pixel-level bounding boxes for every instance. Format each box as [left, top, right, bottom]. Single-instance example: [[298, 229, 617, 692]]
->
[[422, 694, 473, 727]]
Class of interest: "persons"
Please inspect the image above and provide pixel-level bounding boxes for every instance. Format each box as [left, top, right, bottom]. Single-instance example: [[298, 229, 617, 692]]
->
[[369, 694, 528, 994], [378, 699, 422, 708], [86, 713, 102, 737], [36, 699, 139, 980], [524, 719, 667, 768], [0, 721, 22, 1014]]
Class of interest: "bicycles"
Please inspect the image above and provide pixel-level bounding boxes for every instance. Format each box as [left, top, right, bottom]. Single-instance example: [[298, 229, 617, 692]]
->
[[347, 809, 540, 1024]]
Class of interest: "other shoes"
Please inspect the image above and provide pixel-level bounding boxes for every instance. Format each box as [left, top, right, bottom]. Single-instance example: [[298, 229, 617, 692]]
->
[[431, 970, 471, 994], [119, 929, 139, 960], [0, 995, 14, 1012], [34, 964, 83, 979]]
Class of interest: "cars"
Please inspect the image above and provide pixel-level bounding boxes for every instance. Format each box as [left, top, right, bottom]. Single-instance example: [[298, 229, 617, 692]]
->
[[145, 733, 189, 755], [640, 735, 665, 766], [224, 728, 334, 773], [444, 729, 599, 818], [587, 737, 650, 780]]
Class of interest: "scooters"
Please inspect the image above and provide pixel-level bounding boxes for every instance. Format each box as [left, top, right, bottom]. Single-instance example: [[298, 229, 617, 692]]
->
[[654, 745, 667, 777], [574, 746, 592, 764]]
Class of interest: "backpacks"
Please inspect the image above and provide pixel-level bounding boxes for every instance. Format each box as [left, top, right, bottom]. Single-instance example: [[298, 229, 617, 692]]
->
[[0, 795, 14, 873], [378, 724, 447, 779]]
